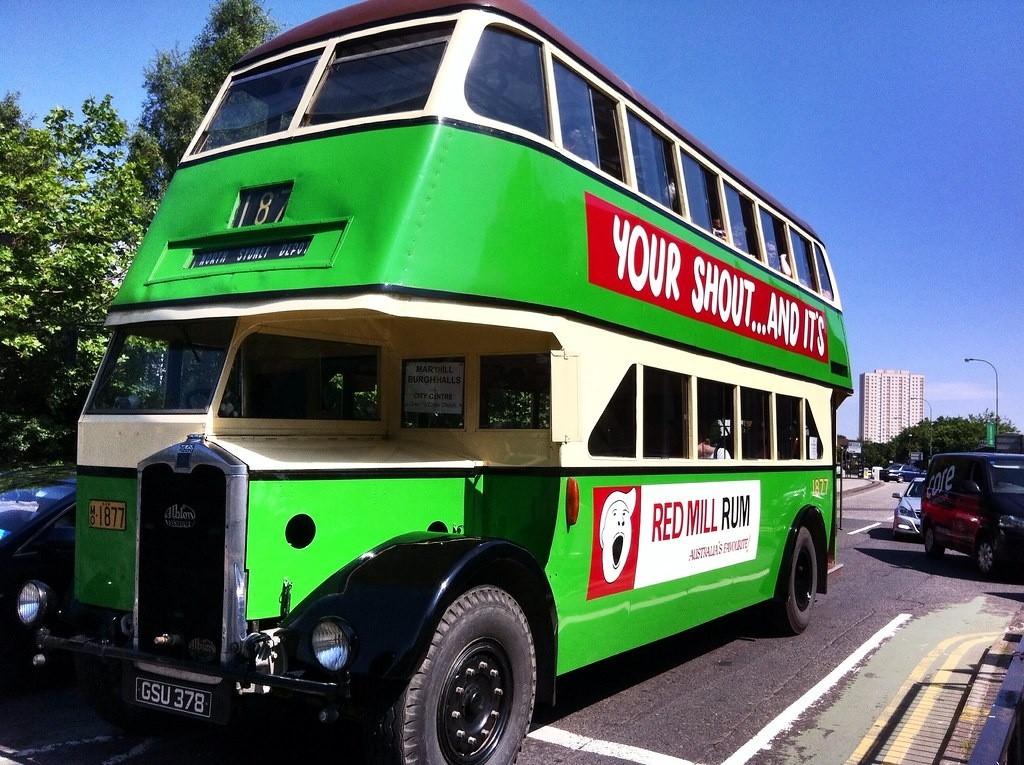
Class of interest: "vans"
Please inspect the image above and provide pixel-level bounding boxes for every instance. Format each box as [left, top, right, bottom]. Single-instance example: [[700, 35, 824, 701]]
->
[[918, 451, 1024, 578]]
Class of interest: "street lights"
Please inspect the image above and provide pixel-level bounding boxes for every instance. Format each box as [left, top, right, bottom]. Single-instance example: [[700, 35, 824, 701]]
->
[[964, 357, 1000, 452], [910, 397, 933, 455]]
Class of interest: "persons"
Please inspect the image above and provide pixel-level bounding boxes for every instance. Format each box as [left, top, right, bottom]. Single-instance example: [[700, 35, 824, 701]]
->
[[698, 438, 732, 460], [711, 208, 723, 230], [780, 254, 792, 276]]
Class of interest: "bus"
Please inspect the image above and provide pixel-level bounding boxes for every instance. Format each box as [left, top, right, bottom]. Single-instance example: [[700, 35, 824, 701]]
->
[[19, 0, 854, 765]]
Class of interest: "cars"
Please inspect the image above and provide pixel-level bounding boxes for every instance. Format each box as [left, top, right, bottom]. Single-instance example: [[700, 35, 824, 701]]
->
[[879, 463, 927, 483], [892, 477, 927, 541], [0, 482, 80, 699]]
